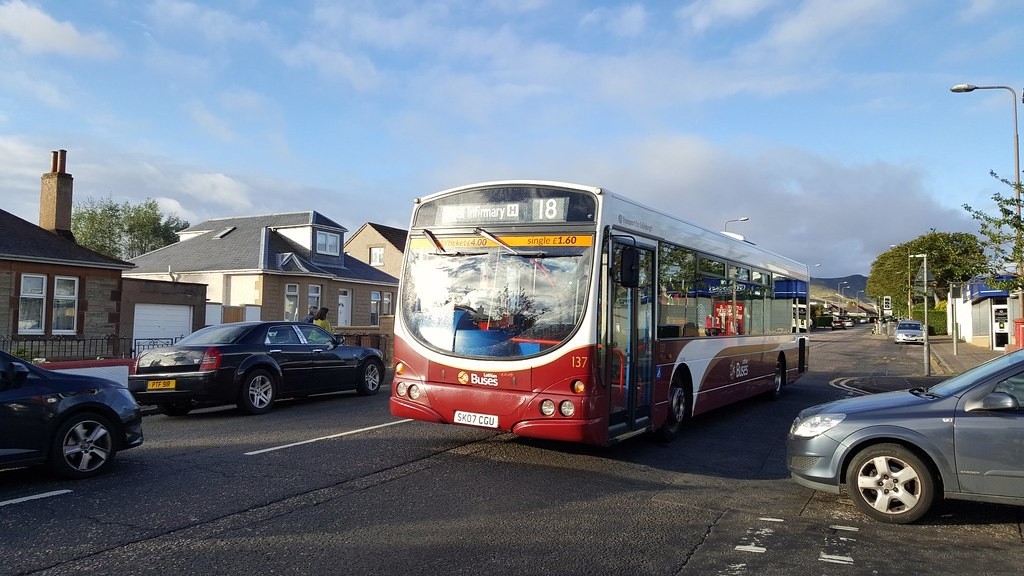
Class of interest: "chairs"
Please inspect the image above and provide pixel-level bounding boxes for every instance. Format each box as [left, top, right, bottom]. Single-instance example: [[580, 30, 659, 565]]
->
[[291, 331, 305, 342], [273, 330, 289, 344]]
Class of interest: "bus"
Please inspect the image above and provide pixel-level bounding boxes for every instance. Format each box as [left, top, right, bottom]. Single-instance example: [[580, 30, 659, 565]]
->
[[389, 180, 809, 444]]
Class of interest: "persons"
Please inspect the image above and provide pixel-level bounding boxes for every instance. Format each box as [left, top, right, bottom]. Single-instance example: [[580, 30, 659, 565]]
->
[[302, 306, 333, 344]]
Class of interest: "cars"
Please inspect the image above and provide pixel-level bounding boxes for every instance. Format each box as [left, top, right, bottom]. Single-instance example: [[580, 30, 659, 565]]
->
[[855, 318, 867, 323], [0, 353, 144, 480], [832, 320, 846, 330], [844, 320, 854, 327], [878, 318, 890, 324], [893, 320, 925, 344], [127, 320, 385, 418], [706, 327, 737, 336], [787, 348, 1024, 524]]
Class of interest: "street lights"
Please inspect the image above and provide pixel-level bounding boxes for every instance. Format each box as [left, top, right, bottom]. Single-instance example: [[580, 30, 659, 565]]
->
[[838, 281, 847, 316], [910, 254, 931, 377], [857, 290, 864, 318], [724, 217, 748, 231], [842, 286, 851, 317], [890, 245, 911, 320], [951, 83, 1024, 321]]
